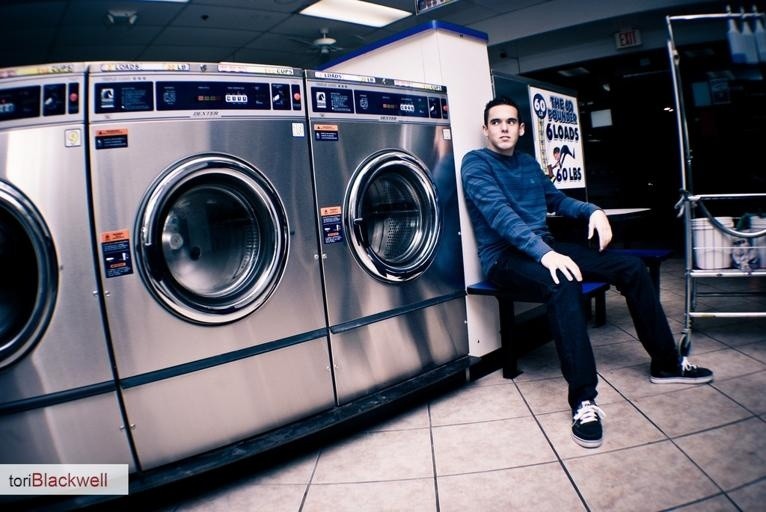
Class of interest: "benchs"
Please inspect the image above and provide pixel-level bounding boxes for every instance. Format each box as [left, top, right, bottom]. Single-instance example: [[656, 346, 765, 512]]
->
[[467, 250, 671, 379]]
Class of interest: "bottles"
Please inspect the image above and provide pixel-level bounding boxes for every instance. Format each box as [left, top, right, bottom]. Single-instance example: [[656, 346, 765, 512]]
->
[[751, 5, 766, 63], [739, 6, 759, 64], [725, 6, 747, 65]]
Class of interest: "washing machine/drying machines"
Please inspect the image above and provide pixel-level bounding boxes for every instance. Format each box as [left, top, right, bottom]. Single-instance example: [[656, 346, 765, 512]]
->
[[305, 68, 469, 407], [1, 62, 140, 495], [85, 61, 335, 471]]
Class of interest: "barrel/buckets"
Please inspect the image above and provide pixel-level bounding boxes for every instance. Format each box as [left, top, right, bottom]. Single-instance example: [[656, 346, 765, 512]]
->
[[693, 217, 734, 269], [749, 217, 765, 270]]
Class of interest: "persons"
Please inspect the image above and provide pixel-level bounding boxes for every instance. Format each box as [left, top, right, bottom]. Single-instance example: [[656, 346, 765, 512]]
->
[[461, 95, 715, 448]]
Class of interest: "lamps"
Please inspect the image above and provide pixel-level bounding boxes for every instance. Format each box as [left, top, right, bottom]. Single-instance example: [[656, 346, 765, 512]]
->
[[297, 0, 412, 28]]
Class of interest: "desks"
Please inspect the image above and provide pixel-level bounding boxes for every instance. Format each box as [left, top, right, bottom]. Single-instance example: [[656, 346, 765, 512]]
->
[[546, 208, 652, 326]]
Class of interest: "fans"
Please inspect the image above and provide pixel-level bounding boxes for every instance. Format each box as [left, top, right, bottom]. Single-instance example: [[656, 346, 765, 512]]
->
[[286, 28, 359, 53]]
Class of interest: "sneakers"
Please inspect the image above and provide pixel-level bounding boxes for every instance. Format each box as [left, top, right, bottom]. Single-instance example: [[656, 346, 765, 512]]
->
[[571, 401, 603, 448], [651, 361, 714, 384]]
